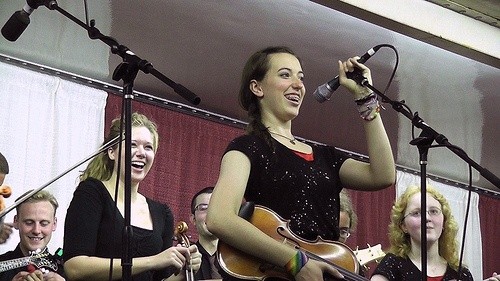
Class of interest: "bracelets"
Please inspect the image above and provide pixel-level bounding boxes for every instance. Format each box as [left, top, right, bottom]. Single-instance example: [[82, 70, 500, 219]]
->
[[284, 250, 309, 277], [353, 93, 382, 121]]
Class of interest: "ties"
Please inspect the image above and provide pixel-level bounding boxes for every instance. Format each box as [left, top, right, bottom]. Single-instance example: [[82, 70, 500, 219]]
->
[[208, 255, 222, 279]]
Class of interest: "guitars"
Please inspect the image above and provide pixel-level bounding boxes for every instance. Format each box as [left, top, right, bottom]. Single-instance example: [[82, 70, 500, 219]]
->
[[352, 239, 387, 266], [1, 252, 56, 273]]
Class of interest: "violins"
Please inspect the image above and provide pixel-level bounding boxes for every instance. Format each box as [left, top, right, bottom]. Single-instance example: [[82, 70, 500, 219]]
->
[[217, 204, 369, 281], [174, 218, 201, 281]]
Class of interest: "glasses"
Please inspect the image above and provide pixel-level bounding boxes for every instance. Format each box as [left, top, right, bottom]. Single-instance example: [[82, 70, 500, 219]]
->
[[340, 230, 351, 239], [400, 208, 441, 221], [194, 203, 208, 213]]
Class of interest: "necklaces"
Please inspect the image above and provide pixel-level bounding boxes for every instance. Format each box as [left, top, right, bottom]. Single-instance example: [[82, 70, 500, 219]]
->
[[268, 128, 298, 146]]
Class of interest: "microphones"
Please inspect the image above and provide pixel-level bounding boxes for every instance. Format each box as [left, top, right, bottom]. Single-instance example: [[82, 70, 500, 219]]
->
[[1, 0, 41, 42], [313, 45, 380, 103]]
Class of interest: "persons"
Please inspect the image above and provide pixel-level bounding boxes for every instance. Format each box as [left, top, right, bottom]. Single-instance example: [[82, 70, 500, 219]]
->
[[0, 154, 15, 245], [61, 112, 203, 281], [188, 185, 223, 280], [369, 183, 500, 281], [204, 44, 396, 281], [339, 190, 357, 242], [0, 188, 69, 281]]
